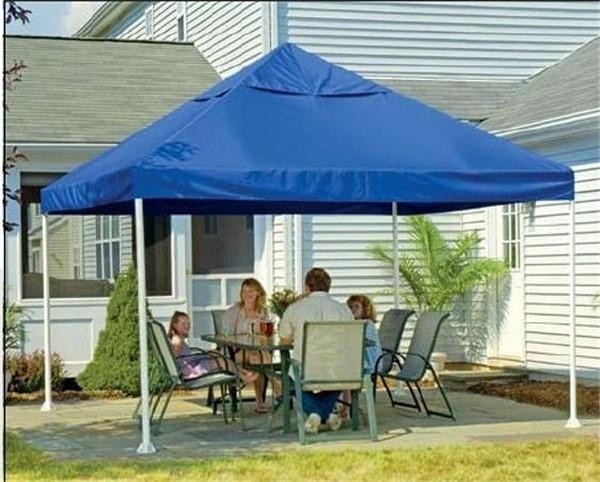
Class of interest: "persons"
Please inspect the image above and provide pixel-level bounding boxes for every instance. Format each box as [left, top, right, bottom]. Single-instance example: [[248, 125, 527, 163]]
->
[[166, 309, 260, 387], [216, 278, 275, 413], [340, 294, 385, 420], [278, 267, 356, 435]]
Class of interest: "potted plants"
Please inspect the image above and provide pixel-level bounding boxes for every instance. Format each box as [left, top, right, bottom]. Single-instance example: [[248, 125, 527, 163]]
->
[[370, 213, 509, 382]]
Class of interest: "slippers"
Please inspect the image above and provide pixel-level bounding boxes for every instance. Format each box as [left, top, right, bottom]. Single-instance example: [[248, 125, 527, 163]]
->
[[255, 403, 267, 414]]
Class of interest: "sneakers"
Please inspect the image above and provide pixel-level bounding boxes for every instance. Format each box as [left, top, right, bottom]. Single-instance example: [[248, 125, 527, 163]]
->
[[304, 413, 321, 434], [326, 413, 343, 431]]
